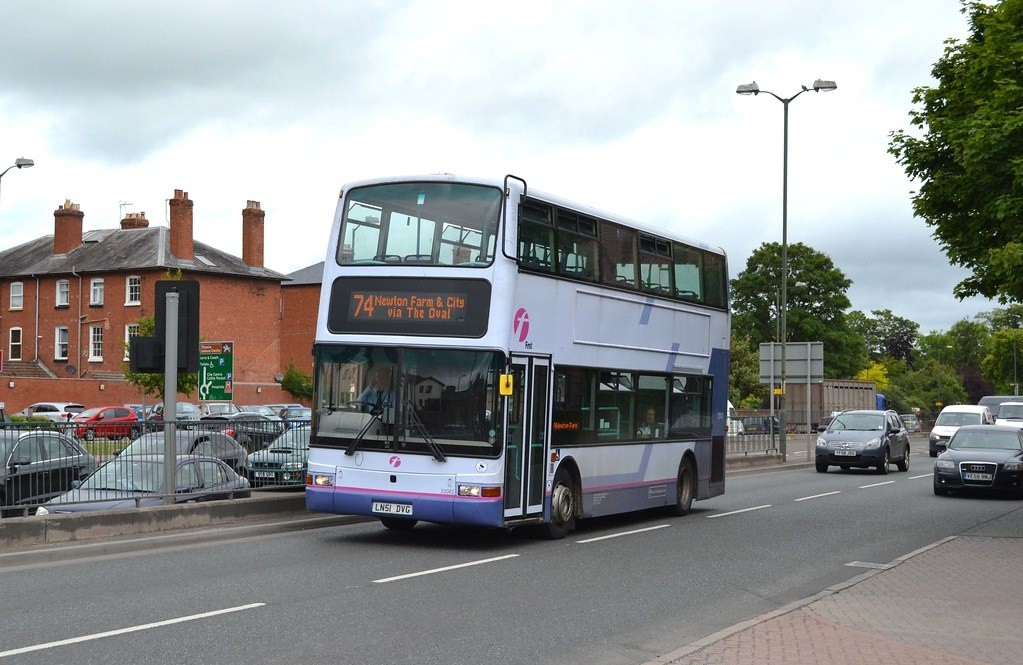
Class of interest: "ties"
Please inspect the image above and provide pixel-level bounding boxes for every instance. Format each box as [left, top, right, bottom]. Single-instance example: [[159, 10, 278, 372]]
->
[[370, 391, 383, 413]]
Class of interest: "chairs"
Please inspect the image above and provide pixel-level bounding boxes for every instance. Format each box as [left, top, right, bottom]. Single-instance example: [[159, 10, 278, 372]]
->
[[475, 255, 493, 264], [519, 256, 698, 301], [372, 255, 433, 266]]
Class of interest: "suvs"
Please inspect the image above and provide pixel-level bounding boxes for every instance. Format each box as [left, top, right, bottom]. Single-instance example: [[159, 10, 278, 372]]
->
[[815, 410, 910, 474]]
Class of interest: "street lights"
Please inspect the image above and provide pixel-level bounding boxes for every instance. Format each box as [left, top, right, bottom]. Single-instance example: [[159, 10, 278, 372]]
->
[[0, 157, 34, 177], [736, 78, 837, 462], [1010, 383, 1018, 395]]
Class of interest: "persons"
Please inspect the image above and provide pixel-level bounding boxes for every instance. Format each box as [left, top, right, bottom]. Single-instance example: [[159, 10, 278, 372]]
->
[[357, 370, 395, 414], [637, 407, 657, 434]]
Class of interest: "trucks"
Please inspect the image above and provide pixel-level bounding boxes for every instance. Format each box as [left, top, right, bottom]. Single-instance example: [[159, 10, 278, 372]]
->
[[726, 400, 745, 437]]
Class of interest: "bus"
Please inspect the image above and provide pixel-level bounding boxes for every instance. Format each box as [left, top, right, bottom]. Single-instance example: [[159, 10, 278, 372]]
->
[[305, 171, 731, 540]]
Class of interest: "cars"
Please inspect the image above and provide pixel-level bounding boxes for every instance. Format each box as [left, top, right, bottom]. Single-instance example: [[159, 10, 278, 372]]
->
[[147, 402, 205, 431], [20, 402, 86, 433], [266, 404, 305, 418], [133, 406, 153, 422], [281, 407, 312, 429], [73, 406, 140, 440], [934, 424, 1023, 498], [819, 417, 835, 434], [198, 403, 240, 415], [246, 426, 310, 486], [899, 414, 921, 432], [199, 412, 285, 455], [36, 454, 251, 515], [929, 405, 994, 457], [112, 430, 250, 479], [0, 429, 98, 518], [994, 402, 1023, 429], [978, 396, 1023, 420], [237, 405, 285, 431]]
